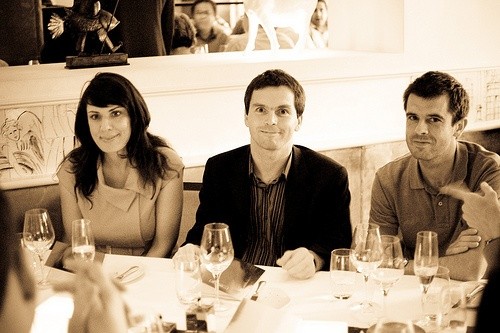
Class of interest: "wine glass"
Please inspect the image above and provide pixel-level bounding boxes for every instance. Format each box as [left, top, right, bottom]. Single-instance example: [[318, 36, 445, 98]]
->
[[199, 222, 235, 312], [407, 231, 439, 326], [22, 208, 56, 289], [349, 221, 406, 318]]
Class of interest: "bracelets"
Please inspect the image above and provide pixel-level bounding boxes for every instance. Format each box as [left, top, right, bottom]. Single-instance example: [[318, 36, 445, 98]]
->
[[483, 237, 500, 247]]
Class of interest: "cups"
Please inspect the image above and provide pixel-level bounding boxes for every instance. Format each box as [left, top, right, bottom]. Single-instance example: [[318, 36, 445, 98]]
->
[[70, 218, 96, 263], [329, 248, 355, 301], [419, 267, 469, 333], [172, 254, 203, 305]]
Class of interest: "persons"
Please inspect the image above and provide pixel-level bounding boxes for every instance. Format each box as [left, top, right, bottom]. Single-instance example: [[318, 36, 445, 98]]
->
[[437, 178, 500, 333], [366, 71, 500, 281], [178, 70, 352, 280], [0, 185, 134, 333], [308, 0, 338, 49], [56, 73, 183, 258], [171, 0, 300, 55]]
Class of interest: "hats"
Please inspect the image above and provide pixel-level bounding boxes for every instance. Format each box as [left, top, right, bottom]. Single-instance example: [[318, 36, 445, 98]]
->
[[243, 0, 280, 50]]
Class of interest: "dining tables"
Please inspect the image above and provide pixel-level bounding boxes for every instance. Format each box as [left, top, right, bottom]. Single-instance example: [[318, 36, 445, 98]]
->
[[24, 239, 485, 333]]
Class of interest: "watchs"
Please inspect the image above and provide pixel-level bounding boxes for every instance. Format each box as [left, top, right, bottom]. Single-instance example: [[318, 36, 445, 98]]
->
[[396, 259, 410, 271]]
